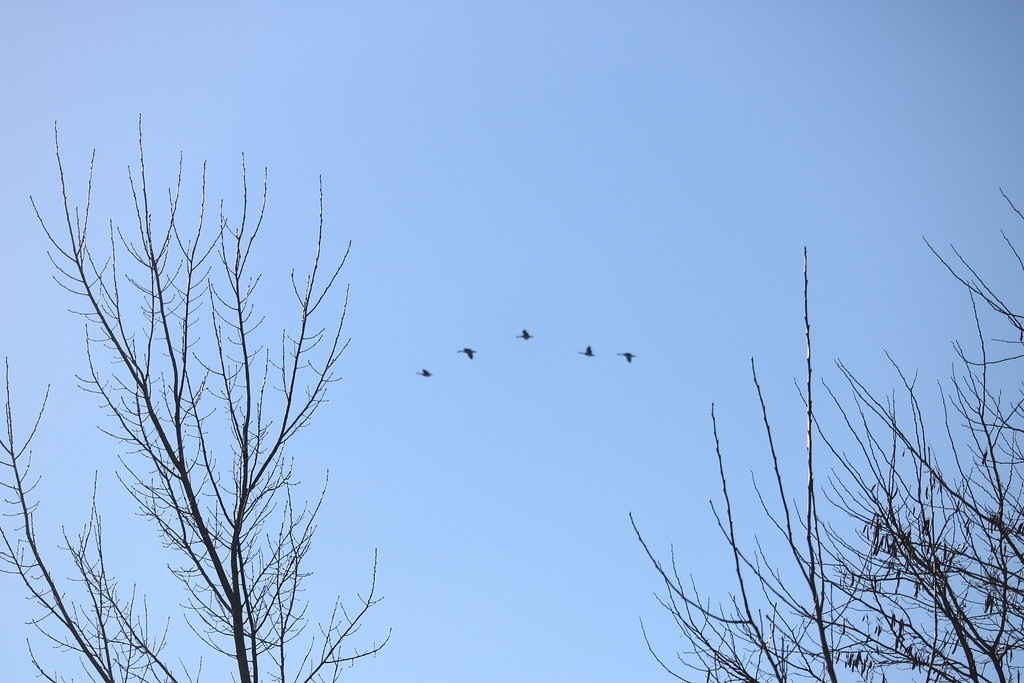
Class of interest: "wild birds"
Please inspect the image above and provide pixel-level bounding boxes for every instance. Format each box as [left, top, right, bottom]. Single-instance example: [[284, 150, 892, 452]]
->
[[457, 348, 476, 360], [416, 369, 434, 377], [616, 353, 636, 364], [578, 345, 596, 357], [516, 329, 534, 340]]
